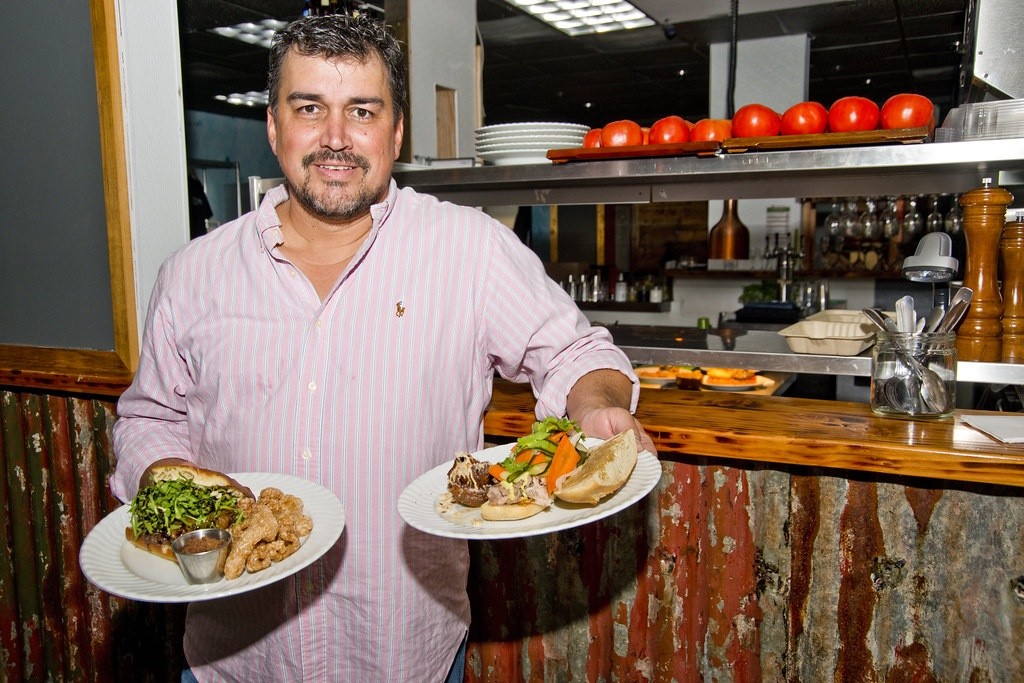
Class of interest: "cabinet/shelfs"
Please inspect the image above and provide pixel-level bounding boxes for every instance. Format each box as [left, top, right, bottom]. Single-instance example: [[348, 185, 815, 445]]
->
[[191, 128, 1024, 416]]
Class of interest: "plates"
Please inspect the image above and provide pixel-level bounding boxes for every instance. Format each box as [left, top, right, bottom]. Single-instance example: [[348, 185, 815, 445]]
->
[[698, 375, 776, 391], [472, 120, 592, 165], [396, 437, 662, 540], [80, 473, 346, 603], [635, 366, 681, 385]]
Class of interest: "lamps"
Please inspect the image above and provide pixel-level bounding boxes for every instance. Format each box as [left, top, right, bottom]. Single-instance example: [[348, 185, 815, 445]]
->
[[901, 231, 959, 314]]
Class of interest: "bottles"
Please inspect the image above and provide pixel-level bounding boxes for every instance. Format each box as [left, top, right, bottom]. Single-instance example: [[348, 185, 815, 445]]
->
[[709, 198, 751, 261], [557, 271, 673, 303], [763, 230, 827, 316]]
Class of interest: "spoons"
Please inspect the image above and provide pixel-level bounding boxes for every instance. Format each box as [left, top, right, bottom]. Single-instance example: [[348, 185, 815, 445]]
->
[[862, 287, 973, 415]]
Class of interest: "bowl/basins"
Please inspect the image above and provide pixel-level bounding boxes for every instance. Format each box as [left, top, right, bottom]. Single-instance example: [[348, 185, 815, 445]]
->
[[675, 376, 701, 390], [172, 528, 233, 586]]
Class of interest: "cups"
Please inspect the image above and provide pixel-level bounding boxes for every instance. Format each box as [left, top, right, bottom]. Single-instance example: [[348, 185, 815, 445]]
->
[[869, 333, 957, 419]]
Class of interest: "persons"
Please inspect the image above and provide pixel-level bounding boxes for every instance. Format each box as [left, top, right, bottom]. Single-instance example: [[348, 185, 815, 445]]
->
[[114, 10, 657, 682]]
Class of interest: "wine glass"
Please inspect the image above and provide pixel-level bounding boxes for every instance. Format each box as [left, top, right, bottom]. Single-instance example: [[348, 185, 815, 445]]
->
[[883, 195, 899, 238], [825, 196, 880, 239], [927, 194, 943, 233], [943, 195, 964, 234], [903, 195, 923, 233]]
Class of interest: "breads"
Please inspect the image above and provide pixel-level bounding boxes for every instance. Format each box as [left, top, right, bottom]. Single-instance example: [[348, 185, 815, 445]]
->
[[707, 368, 757, 386]]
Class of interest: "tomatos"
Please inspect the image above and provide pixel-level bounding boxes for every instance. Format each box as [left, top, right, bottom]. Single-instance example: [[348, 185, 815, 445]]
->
[[583, 92, 935, 148]]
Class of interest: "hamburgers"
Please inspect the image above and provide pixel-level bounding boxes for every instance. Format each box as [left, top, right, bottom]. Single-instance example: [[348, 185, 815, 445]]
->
[[479, 416, 638, 521], [126, 456, 258, 566]]
